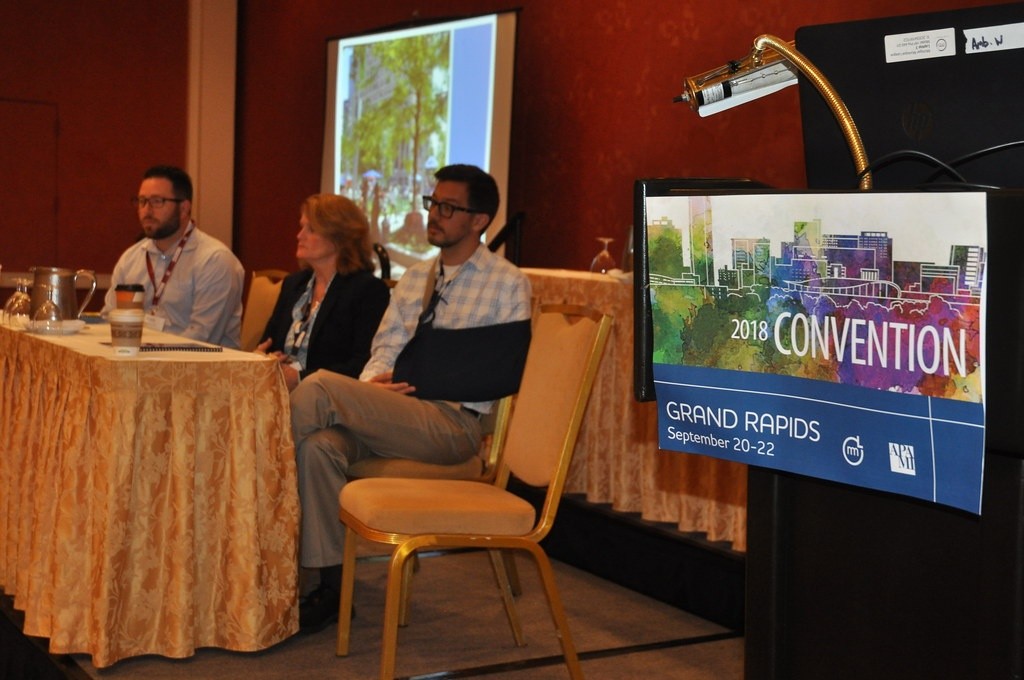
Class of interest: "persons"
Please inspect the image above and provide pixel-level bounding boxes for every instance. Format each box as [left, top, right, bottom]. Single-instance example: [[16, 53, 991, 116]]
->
[[287, 164, 532, 632], [100, 164, 246, 351], [254, 193, 391, 393]]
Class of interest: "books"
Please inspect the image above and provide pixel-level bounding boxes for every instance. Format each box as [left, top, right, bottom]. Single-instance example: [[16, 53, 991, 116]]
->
[[141, 343, 223, 352]]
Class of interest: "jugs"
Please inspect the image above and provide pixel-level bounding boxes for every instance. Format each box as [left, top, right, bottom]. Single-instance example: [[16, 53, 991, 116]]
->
[[29, 266, 97, 321]]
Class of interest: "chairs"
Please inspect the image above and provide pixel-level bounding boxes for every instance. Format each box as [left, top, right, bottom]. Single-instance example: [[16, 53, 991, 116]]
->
[[335, 295, 613, 680], [239, 270, 289, 354]]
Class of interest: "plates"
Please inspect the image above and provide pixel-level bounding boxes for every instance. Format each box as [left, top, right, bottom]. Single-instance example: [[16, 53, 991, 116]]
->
[[24, 320, 85, 334]]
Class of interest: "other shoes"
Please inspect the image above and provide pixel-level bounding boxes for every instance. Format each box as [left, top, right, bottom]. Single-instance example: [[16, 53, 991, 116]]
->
[[299, 581, 356, 634]]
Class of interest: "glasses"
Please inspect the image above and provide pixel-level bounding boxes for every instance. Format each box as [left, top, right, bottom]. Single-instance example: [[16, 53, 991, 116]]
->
[[423, 194, 483, 218], [137, 195, 184, 209]]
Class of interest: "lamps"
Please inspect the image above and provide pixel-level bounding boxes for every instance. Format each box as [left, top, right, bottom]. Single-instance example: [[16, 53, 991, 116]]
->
[[674, 34, 872, 189]]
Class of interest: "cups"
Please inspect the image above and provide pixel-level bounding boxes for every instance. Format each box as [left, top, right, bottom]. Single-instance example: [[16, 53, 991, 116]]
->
[[114, 283, 145, 310], [108, 308, 144, 356]]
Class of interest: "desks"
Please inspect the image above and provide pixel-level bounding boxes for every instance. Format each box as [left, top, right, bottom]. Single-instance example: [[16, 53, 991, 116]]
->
[[0, 312, 302, 669], [508, 262, 746, 553]]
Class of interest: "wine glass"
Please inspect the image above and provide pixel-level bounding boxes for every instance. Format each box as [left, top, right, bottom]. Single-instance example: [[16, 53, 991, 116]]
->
[[10, 286, 31, 328], [3, 278, 26, 325], [33, 285, 62, 321]]
